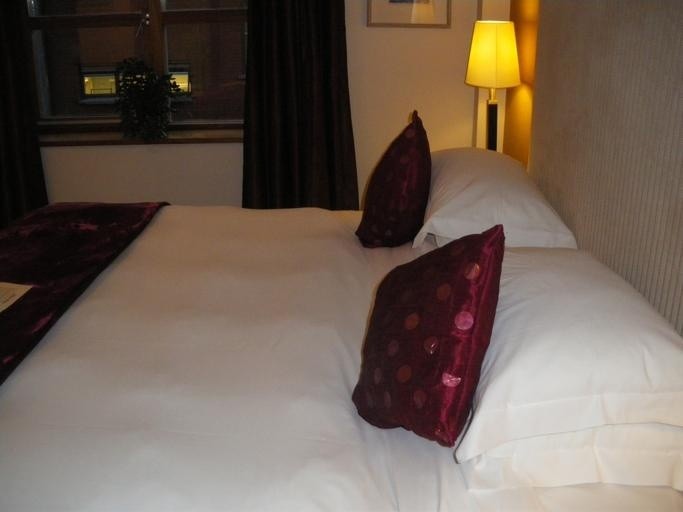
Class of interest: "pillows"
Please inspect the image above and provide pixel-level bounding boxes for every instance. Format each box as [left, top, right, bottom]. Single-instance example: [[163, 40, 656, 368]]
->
[[454, 248, 683, 465], [412, 147, 578, 252], [455, 421, 683, 493], [352, 222, 506, 447], [354, 109, 432, 249]]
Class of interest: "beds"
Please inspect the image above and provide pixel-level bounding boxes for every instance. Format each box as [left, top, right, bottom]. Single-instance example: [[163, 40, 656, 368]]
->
[[0, 201, 683, 512]]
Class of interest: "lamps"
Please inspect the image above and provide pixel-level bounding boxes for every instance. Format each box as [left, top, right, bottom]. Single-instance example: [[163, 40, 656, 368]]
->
[[464, 20, 521, 151]]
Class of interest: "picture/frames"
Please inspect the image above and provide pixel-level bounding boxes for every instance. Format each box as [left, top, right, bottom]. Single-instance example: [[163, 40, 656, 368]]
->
[[367, 0, 452, 30]]
